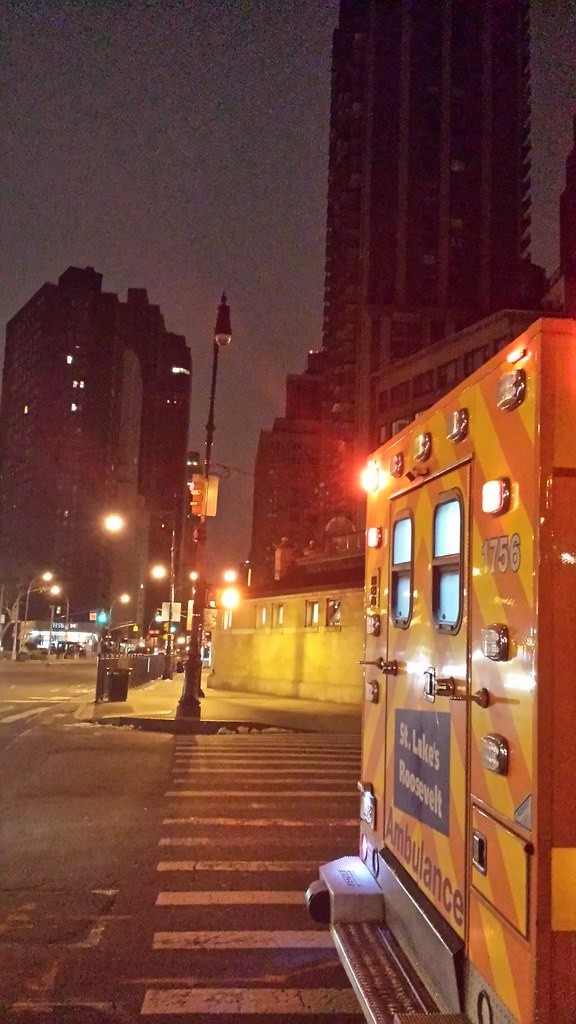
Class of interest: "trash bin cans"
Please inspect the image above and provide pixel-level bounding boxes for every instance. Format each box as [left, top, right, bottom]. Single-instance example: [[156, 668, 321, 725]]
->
[[106, 667, 133, 702]]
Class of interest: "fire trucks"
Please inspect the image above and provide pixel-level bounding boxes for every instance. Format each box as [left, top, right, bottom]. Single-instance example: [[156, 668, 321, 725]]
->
[[302, 316, 576, 1023]]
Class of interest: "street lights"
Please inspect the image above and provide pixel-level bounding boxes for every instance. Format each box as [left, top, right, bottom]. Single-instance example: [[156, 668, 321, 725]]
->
[[22, 570, 52, 652], [106, 595, 129, 655], [51, 586, 70, 648], [173, 295, 235, 721]]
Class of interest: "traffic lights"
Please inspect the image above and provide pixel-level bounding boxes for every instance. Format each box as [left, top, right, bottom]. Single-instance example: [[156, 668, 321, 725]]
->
[[98, 613, 106, 623], [172, 626, 177, 632]]
